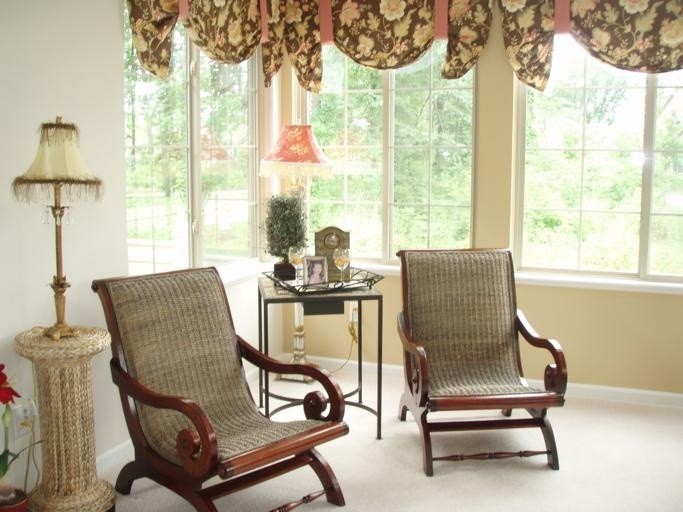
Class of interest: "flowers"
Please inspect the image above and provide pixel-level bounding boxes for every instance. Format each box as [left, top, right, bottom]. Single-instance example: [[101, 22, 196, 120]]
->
[[0, 362, 44, 501]]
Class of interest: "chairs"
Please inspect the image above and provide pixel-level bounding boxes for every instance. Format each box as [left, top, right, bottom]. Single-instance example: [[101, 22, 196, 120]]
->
[[91, 267, 349, 512], [395, 248, 568, 476]]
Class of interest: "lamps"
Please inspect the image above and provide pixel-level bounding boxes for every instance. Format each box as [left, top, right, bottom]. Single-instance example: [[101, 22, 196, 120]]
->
[[12, 116, 102, 341], [261, 122, 333, 383]]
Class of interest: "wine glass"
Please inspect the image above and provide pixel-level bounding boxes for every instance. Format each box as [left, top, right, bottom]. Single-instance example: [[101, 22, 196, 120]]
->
[[288, 245, 307, 288], [332, 247, 351, 287]]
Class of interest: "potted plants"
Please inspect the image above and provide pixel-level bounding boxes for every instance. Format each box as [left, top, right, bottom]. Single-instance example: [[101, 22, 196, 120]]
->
[[264, 192, 307, 280]]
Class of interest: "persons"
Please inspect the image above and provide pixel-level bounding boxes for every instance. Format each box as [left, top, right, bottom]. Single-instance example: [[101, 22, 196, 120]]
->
[[307, 261, 324, 283]]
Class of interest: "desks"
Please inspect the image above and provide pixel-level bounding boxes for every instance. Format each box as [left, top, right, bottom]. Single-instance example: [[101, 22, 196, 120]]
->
[[258, 275, 382, 439]]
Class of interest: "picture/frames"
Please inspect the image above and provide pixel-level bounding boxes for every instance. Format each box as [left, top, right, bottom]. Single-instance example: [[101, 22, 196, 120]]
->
[[303, 256, 330, 286]]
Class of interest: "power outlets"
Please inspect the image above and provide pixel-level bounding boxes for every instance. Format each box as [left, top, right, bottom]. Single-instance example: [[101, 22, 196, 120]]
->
[[11, 405, 35, 438], [347, 301, 359, 322]]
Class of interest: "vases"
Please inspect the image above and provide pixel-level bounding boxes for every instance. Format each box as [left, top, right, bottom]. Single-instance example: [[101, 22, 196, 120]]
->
[[0, 487, 28, 512]]
